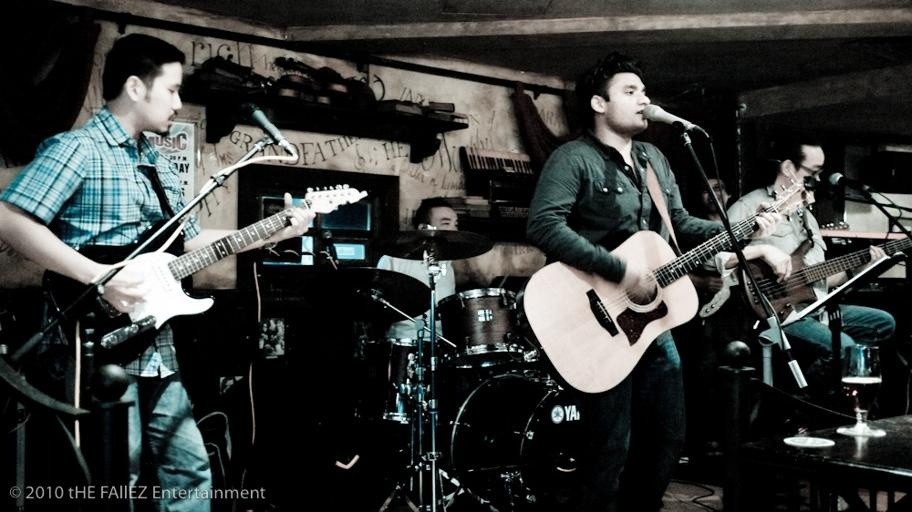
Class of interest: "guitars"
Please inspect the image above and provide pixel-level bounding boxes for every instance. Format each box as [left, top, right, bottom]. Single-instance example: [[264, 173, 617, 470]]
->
[[516, 179, 811, 396], [62, 183, 370, 367], [742, 233, 911, 321]]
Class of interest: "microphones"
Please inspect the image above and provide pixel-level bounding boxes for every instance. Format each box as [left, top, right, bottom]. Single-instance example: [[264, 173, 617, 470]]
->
[[247, 103, 293, 157], [830, 172, 873, 193], [642, 104, 696, 133], [514, 467, 536, 504], [322, 230, 339, 265]]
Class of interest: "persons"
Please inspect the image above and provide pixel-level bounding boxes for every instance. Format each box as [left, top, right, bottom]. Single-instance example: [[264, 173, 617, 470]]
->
[[695, 170, 732, 229], [725, 138, 896, 381], [0, 33, 320, 510], [375, 196, 460, 354], [525, 54, 784, 511]]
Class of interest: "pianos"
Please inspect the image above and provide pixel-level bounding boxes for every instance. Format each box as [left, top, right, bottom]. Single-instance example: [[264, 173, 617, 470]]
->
[[459, 144, 537, 181]]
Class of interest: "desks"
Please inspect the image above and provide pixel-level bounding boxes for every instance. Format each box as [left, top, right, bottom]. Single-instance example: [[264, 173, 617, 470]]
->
[[780, 415, 912, 511]]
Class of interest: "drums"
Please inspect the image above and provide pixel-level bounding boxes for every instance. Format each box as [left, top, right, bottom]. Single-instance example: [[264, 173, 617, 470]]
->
[[382, 288, 583, 512]]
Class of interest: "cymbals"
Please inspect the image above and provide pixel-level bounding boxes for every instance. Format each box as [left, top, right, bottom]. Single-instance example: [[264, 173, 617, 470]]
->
[[287, 267, 430, 324], [371, 229, 493, 260]]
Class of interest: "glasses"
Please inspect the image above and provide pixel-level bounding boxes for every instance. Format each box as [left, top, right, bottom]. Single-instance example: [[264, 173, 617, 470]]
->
[[799, 164, 824, 177]]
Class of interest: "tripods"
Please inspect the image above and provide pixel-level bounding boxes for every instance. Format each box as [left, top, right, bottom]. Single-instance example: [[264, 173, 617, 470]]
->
[[356, 244, 498, 512]]
